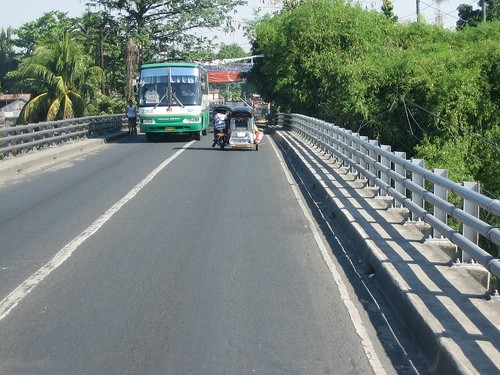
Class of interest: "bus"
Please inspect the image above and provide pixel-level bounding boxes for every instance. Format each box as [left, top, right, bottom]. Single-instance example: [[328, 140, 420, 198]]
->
[[133, 61, 211, 141]]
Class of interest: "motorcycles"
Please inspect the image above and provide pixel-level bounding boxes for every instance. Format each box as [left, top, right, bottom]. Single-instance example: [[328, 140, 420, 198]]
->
[[213, 108, 259, 150]]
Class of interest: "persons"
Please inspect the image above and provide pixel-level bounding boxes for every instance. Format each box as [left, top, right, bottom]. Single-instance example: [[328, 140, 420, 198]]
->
[[212, 109, 227, 147], [126, 100, 137, 132], [144, 85, 159, 102], [184, 87, 195, 97]]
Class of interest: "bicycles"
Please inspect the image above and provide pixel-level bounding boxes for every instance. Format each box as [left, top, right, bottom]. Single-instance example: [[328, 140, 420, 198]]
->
[[126, 115, 137, 137]]
